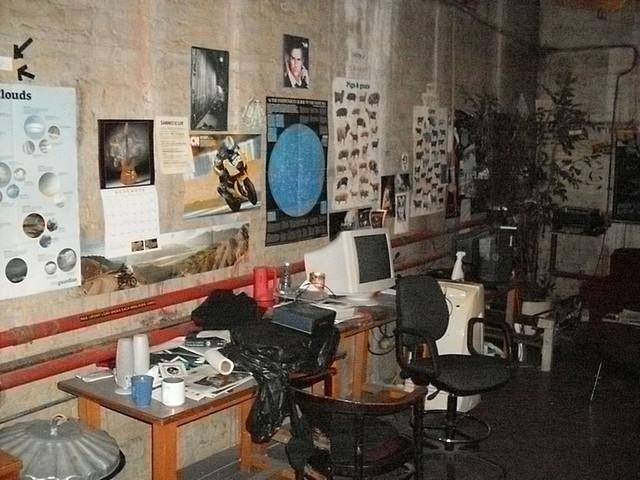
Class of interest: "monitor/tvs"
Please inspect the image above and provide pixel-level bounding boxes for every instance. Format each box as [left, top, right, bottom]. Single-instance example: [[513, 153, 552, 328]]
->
[[613, 136, 640, 223], [303, 227, 397, 307]]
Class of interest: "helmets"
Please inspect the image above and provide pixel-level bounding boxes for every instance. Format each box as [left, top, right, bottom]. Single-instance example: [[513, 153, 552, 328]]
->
[[224, 136, 234, 150]]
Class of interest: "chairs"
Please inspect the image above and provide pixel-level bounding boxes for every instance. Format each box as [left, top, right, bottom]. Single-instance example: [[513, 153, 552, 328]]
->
[[285, 367, 432, 480], [581, 247, 640, 403], [394, 275, 518, 452]]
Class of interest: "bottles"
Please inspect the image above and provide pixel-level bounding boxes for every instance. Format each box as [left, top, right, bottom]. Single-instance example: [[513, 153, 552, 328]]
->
[[281, 262, 292, 293]]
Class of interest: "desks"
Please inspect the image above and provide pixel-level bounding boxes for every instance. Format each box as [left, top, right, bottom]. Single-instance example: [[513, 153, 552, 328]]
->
[[56, 339, 338, 480]]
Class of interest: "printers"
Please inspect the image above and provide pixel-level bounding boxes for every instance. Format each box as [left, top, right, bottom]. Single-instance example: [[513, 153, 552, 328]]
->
[[555, 205, 601, 231]]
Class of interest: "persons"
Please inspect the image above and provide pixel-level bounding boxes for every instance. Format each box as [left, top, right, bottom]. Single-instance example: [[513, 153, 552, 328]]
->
[[454, 141, 484, 195], [212, 135, 242, 191], [285, 42, 311, 88]]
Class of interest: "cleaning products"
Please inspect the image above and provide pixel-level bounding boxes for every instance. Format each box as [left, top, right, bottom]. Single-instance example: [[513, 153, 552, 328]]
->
[[452, 250, 467, 281]]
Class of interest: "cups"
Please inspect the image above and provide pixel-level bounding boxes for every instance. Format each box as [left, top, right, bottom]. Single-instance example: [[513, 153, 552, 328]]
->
[[131, 375, 154, 405], [253, 266, 277, 300], [161, 377, 185, 406]]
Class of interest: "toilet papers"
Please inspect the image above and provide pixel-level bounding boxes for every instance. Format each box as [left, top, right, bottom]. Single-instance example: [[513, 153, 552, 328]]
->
[[208, 349, 235, 376]]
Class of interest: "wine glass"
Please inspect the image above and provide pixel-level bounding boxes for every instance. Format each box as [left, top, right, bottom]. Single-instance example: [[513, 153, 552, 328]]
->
[[115, 335, 150, 397]]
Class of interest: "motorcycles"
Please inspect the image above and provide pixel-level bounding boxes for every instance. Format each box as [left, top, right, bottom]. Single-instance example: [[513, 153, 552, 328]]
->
[[211, 143, 256, 213], [113, 269, 138, 289]]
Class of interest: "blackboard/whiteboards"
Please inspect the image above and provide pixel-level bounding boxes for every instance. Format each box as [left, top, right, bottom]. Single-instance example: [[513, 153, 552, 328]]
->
[[610, 146, 639, 225]]
